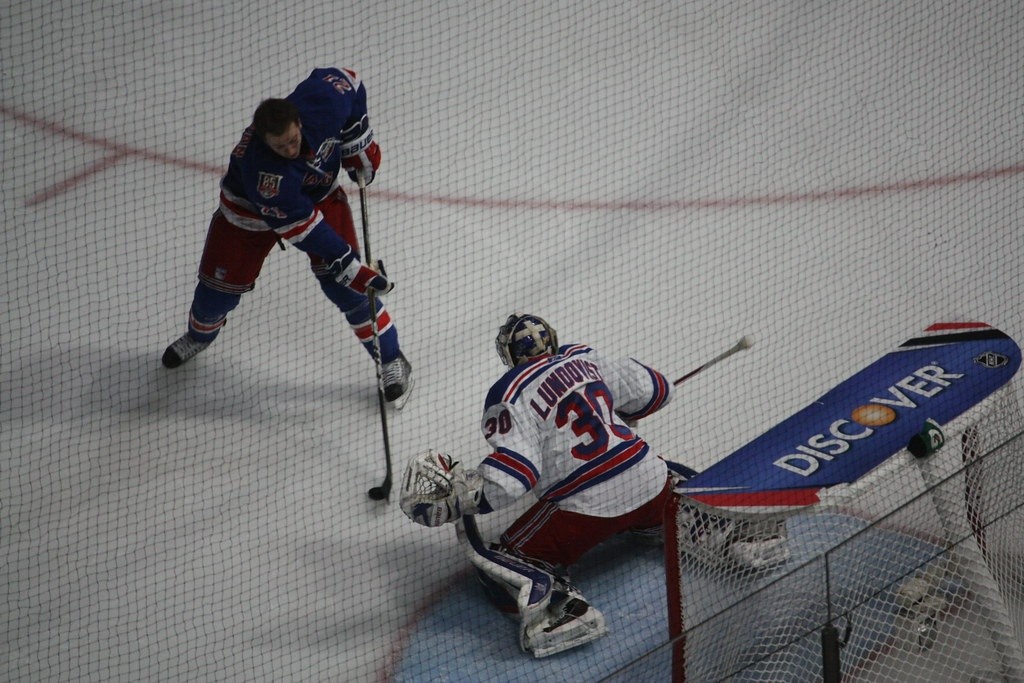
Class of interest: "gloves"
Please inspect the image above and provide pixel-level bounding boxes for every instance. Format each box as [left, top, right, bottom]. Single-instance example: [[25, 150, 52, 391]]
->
[[340, 114, 382, 185], [326, 244, 395, 297]]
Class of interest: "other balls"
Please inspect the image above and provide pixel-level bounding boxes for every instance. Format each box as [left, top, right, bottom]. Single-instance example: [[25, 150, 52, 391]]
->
[[368, 486, 386, 501]]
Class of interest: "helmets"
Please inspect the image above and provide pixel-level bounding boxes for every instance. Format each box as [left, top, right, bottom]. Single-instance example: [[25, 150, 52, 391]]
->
[[496, 313, 559, 366]]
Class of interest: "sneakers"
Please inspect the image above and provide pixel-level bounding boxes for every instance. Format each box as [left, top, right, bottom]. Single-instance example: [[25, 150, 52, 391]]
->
[[382, 350, 415, 409], [525, 594, 607, 658], [724, 532, 792, 571], [161, 332, 211, 369]]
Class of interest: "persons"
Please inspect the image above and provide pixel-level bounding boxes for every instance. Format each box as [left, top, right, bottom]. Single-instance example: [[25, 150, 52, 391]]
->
[[399, 313, 791, 659], [161, 67, 417, 411]]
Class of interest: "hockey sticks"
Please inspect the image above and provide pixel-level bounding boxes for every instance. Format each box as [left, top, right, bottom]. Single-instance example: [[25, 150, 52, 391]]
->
[[673, 330, 758, 387], [356, 171, 394, 505]]
